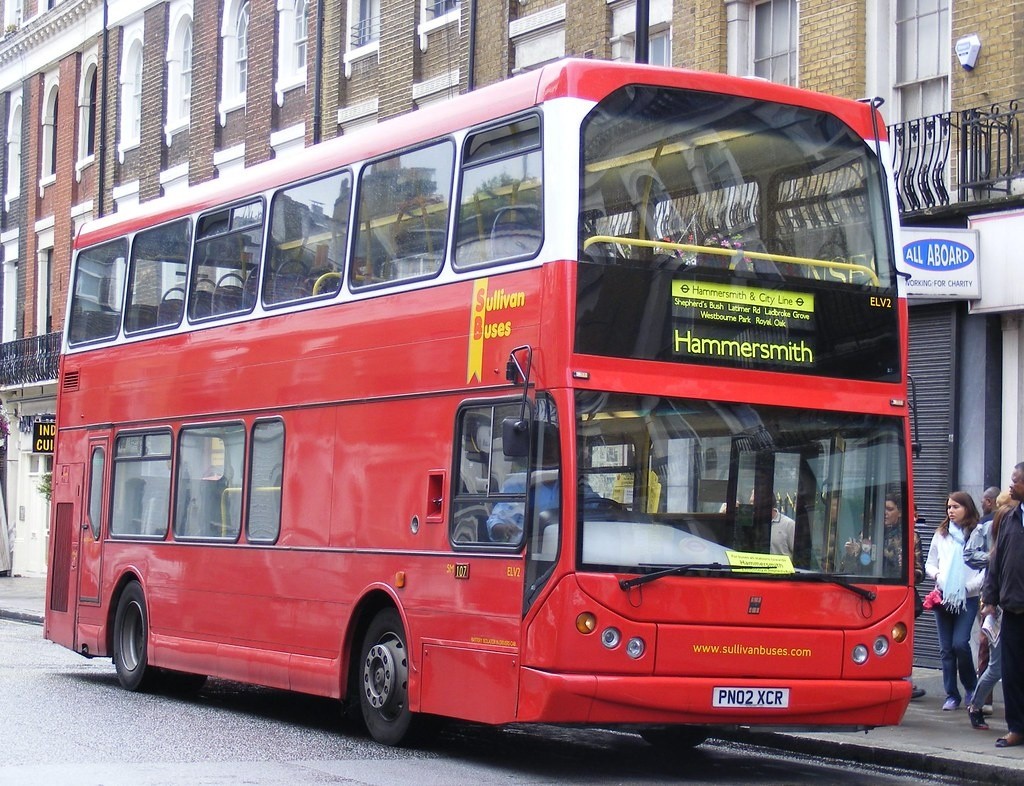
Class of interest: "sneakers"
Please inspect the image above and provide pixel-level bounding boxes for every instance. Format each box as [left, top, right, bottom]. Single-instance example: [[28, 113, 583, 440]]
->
[[942, 697, 959, 711], [965, 704, 988, 730], [908, 685, 927, 698]]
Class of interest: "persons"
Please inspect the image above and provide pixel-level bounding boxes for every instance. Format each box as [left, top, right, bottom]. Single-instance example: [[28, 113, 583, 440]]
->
[[924, 490, 988, 712], [978, 485, 1001, 526], [963, 490, 1020, 715], [846, 493, 927, 584], [485, 456, 603, 545], [981, 461, 1024, 748], [904, 502, 927, 704], [968, 503, 1004, 731], [818, 490, 859, 574], [751, 487, 795, 563]]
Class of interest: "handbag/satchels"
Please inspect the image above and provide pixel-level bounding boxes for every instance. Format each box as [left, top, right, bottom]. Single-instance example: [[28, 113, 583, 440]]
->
[[914, 588, 924, 621]]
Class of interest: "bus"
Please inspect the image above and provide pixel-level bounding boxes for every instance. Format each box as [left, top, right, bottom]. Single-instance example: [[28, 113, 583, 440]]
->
[[42, 40, 920, 754]]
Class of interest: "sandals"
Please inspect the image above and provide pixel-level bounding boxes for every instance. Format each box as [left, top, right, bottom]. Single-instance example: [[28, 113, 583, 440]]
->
[[994, 729, 1023, 747]]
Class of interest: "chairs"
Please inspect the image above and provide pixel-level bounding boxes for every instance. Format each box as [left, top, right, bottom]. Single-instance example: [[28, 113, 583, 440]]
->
[[79, 203, 542, 350], [117, 474, 282, 543]]
